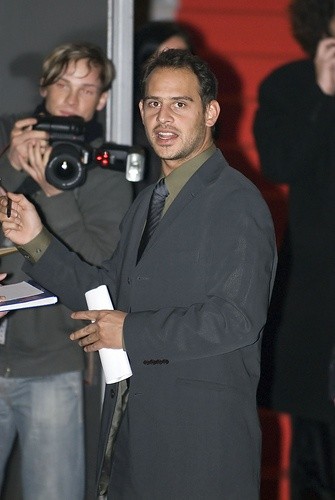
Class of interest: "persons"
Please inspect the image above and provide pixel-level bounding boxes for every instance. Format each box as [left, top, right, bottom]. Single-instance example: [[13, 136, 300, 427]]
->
[[256, 0, 335, 500], [69, 24, 276, 500], [0, 273, 9, 319], [0, 40, 133, 500]]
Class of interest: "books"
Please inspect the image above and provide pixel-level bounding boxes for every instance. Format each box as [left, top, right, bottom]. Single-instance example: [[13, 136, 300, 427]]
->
[[0, 280, 58, 312]]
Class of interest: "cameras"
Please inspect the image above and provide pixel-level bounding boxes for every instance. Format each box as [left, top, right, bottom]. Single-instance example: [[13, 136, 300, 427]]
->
[[28, 114, 144, 188]]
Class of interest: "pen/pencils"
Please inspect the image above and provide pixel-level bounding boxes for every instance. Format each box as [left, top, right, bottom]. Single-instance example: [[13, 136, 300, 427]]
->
[[7, 198, 12, 217]]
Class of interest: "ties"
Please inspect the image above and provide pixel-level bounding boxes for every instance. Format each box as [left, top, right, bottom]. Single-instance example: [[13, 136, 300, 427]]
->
[[137, 176, 168, 264]]
